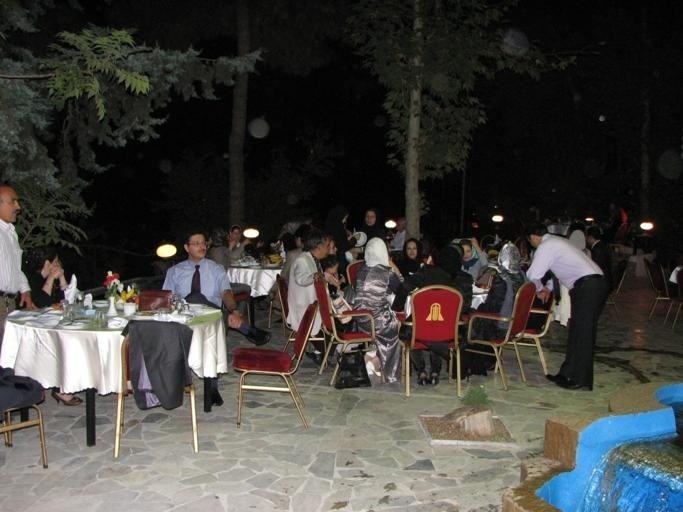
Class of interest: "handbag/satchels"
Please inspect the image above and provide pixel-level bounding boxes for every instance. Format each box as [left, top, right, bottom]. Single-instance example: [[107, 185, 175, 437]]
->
[[139, 288, 172, 312]]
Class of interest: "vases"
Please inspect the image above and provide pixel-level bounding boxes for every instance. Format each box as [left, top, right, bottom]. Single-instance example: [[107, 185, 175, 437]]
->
[[107, 295, 118, 317]]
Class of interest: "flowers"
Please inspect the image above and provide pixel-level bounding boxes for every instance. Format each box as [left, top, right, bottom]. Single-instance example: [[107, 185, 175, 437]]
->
[[103, 270, 120, 296]]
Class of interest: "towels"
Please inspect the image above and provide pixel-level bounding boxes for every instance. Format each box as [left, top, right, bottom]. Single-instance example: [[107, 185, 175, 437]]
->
[[65, 273, 78, 305]]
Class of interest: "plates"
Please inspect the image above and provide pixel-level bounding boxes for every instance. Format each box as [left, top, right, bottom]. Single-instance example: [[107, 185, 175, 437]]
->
[[45, 306, 64, 314], [93, 299, 109, 307], [134, 310, 156, 318], [62, 320, 85, 329]]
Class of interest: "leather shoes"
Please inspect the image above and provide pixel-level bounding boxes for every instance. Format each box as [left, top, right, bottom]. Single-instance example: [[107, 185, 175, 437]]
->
[[245, 328, 271, 345], [306, 352, 331, 366], [417, 377, 428, 386], [428, 377, 438, 385], [546, 371, 567, 382], [557, 376, 592, 391]]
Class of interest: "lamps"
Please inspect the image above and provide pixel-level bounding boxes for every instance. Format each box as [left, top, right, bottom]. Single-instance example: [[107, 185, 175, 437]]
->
[[638, 219, 654, 232], [156, 240, 178, 259], [489, 209, 505, 223], [385, 218, 397, 230], [242, 226, 260, 240], [583, 215, 595, 221]]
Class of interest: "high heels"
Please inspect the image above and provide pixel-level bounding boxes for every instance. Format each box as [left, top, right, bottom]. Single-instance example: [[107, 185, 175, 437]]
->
[[51, 391, 83, 405]]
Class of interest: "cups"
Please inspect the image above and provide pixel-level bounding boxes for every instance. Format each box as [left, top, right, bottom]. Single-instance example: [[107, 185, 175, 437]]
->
[[75, 291, 85, 311], [60, 300, 70, 318], [123, 303, 136, 317], [158, 309, 168, 320], [95, 310, 105, 329]]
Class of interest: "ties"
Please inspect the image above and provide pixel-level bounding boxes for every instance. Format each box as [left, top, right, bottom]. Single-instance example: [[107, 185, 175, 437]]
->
[[192, 265, 200, 294]]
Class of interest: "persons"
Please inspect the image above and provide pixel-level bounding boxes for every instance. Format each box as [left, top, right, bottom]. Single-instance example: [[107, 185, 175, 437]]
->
[[453, 237, 487, 280], [205, 228, 250, 272], [321, 254, 340, 297], [162, 234, 271, 408], [470, 242, 529, 377], [0, 188, 37, 342], [322, 206, 360, 271], [391, 244, 473, 386], [358, 209, 384, 245], [280, 224, 310, 252], [320, 238, 337, 256], [15, 246, 84, 405], [391, 218, 406, 250], [228, 224, 246, 260], [400, 239, 422, 279], [526, 222, 608, 391], [346, 238, 406, 384], [287, 229, 339, 369], [568, 230, 591, 261], [586, 226, 611, 295]]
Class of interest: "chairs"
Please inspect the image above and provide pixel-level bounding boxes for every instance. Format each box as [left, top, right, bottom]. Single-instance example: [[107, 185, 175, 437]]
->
[[232, 300, 320, 430], [228, 283, 251, 327], [494, 295, 557, 377], [463, 281, 536, 391], [397, 285, 462, 399], [345, 260, 365, 289], [276, 273, 330, 370], [114, 319, 199, 457], [641, 259, 674, 321], [601, 259, 629, 326], [313, 272, 386, 385], [662, 295, 683, 328], [0, 366, 50, 470], [136, 289, 173, 310]]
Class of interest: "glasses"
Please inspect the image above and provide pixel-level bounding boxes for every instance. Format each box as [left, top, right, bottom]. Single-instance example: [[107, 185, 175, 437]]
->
[[190, 240, 208, 245]]
